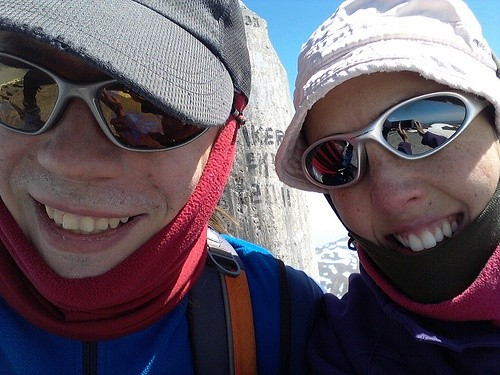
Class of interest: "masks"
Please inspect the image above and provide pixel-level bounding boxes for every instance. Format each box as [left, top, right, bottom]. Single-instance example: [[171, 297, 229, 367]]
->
[[349, 174, 500, 304]]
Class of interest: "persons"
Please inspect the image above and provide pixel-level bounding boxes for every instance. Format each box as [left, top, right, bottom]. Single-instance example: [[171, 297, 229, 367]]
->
[[99, 87, 169, 119], [111, 112, 202, 149], [309, 140, 358, 185], [274, 0, 500, 375], [23, 69, 39, 115], [0, 0, 321, 375], [398, 120, 448, 155]]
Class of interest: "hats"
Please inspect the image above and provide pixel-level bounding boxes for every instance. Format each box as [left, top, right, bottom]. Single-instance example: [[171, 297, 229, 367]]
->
[[0, 0, 251, 125], [275, 1, 500, 192]]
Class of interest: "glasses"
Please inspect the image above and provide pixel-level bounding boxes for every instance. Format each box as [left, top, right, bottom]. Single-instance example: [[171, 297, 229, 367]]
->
[[297, 92, 492, 189], [0, 53, 211, 152]]
